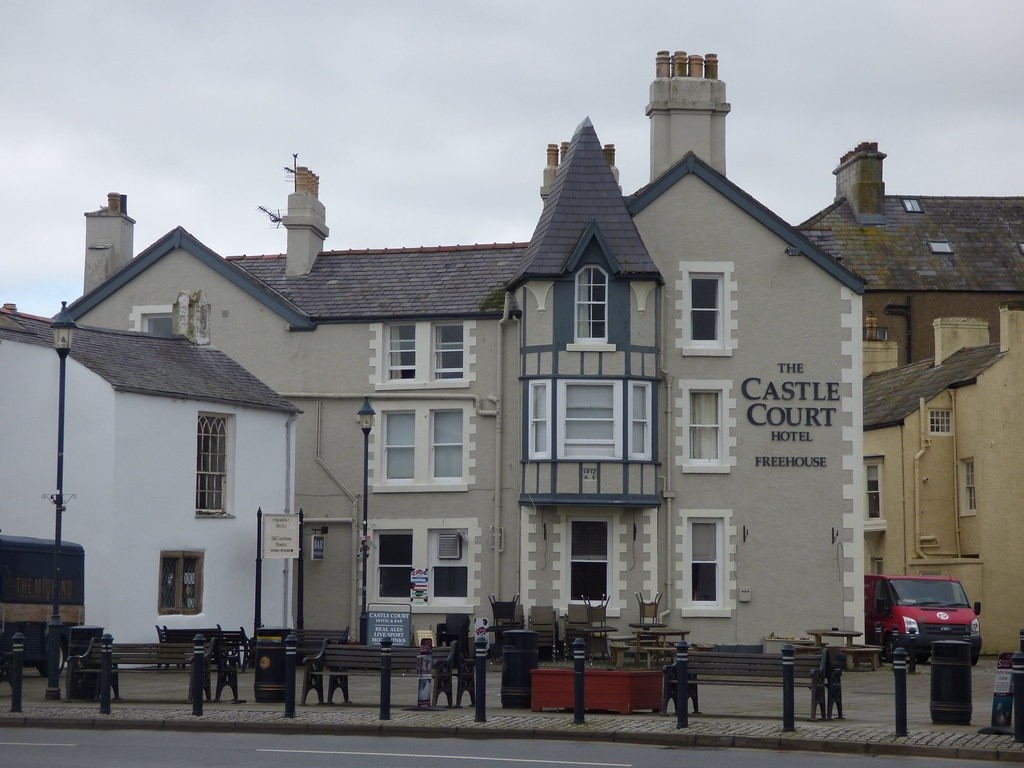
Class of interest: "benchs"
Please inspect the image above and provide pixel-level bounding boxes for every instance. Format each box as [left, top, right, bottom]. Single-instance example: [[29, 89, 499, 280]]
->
[[68, 624, 883, 722]]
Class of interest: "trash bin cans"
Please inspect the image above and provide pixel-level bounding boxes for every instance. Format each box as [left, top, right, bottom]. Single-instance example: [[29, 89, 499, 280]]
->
[[254, 627, 292, 703], [66, 625, 105, 701], [930, 641, 973, 726], [501, 629, 539, 709]]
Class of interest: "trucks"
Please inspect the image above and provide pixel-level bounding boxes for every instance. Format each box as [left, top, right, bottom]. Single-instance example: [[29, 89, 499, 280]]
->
[[1, 533, 87, 675]]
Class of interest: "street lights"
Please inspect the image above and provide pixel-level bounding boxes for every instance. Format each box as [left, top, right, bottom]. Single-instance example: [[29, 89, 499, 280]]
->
[[42, 300, 78, 701], [356, 397, 376, 647]]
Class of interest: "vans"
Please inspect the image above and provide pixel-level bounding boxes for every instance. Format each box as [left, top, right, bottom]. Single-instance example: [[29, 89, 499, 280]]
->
[[865, 574, 984, 667]]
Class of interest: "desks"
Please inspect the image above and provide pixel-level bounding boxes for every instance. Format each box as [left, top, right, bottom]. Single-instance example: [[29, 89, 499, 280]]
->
[[806, 627, 864, 649]]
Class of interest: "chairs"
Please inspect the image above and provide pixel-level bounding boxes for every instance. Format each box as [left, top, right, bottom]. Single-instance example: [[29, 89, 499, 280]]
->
[[488, 590, 662, 660]]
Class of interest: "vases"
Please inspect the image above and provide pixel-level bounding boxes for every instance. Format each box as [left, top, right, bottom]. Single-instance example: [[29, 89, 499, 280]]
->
[[763, 637, 814, 654]]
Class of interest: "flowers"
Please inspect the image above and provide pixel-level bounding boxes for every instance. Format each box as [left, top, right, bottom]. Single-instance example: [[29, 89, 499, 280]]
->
[[769, 632, 809, 640]]
